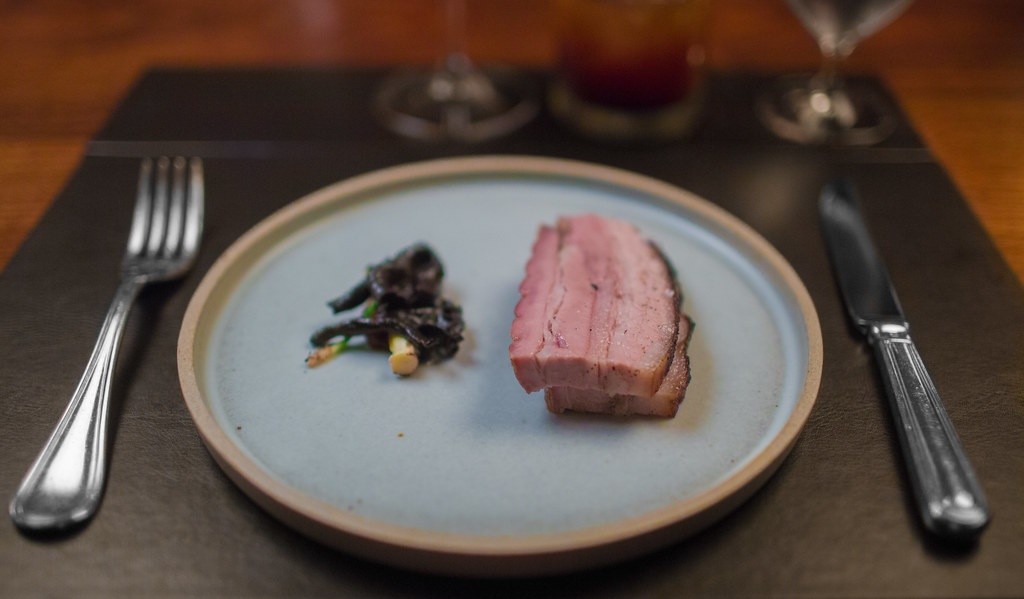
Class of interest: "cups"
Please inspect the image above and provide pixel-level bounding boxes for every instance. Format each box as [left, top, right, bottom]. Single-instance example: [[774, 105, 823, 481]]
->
[[551, 2, 717, 141], [373, 1, 539, 144]]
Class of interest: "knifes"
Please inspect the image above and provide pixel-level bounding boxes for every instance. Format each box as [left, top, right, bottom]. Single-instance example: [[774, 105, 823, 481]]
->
[[820, 173, 988, 534]]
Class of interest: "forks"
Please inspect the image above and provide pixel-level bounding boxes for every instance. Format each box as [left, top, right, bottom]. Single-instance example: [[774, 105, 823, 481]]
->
[[7, 155, 205, 530]]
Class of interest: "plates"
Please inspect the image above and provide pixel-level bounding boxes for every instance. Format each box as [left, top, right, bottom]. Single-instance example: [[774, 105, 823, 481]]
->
[[177, 154, 823, 578]]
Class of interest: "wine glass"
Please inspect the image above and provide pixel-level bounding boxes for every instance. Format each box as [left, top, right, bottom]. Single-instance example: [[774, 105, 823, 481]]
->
[[759, 0, 915, 148]]
